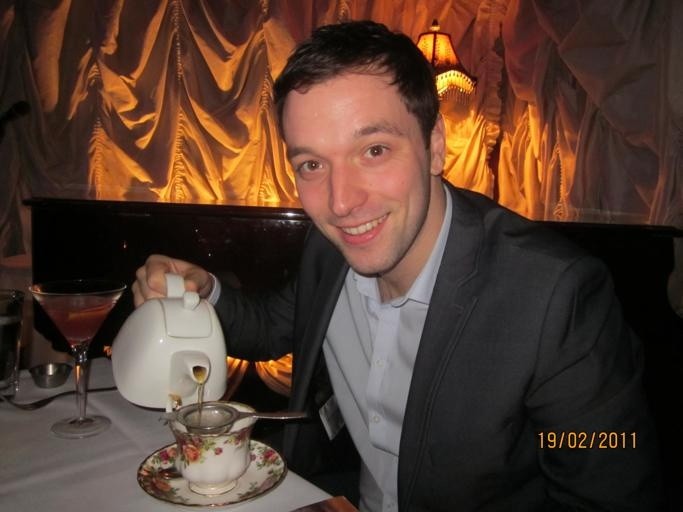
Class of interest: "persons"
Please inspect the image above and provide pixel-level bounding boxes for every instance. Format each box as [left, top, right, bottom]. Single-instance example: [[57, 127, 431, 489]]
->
[[130, 20, 660, 512]]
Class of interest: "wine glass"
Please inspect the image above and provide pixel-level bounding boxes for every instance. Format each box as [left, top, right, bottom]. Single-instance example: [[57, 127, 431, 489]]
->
[[28, 279, 127, 441]]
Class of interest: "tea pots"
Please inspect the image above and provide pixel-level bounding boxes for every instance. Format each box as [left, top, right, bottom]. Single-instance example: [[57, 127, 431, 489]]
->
[[110, 270, 228, 409]]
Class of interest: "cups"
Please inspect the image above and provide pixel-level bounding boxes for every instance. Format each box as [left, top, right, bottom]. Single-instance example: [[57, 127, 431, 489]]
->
[[168, 400, 259, 495], [0, 287, 25, 402]]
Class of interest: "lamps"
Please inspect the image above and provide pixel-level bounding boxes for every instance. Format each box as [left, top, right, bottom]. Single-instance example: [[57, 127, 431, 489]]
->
[[416, 19, 477, 101]]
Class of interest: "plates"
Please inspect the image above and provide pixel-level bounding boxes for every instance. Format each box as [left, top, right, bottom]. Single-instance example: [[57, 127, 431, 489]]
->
[[137, 435, 288, 509]]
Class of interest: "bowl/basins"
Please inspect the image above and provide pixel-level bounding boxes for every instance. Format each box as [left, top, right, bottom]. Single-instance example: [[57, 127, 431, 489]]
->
[[31, 362, 73, 388]]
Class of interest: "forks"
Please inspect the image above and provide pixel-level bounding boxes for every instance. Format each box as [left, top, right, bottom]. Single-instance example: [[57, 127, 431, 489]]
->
[[3, 386, 117, 412]]
